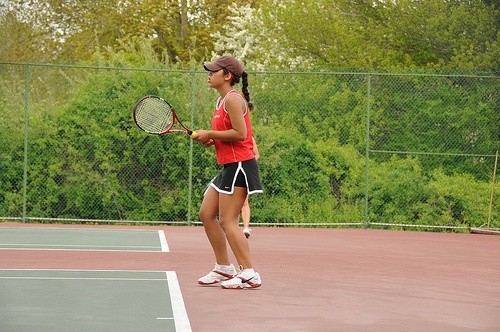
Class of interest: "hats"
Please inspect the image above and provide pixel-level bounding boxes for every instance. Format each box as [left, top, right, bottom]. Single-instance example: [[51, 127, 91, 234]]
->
[[203, 56, 244, 78]]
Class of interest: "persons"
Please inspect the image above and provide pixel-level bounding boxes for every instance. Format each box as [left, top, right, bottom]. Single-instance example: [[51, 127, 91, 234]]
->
[[237, 134, 260, 238], [190, 56, 264, 289]]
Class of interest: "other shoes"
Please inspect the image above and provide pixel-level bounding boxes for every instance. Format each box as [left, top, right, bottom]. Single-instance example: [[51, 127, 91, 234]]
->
[[243, 228, 252, 239]]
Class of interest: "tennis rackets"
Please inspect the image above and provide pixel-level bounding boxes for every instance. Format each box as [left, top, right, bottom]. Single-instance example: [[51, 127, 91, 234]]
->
[[135, 96, 199, 139]]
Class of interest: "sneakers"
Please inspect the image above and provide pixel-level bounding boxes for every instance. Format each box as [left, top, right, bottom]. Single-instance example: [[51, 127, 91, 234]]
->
[[197, 264, 237, 285], [221, 265, 262, 289]]
